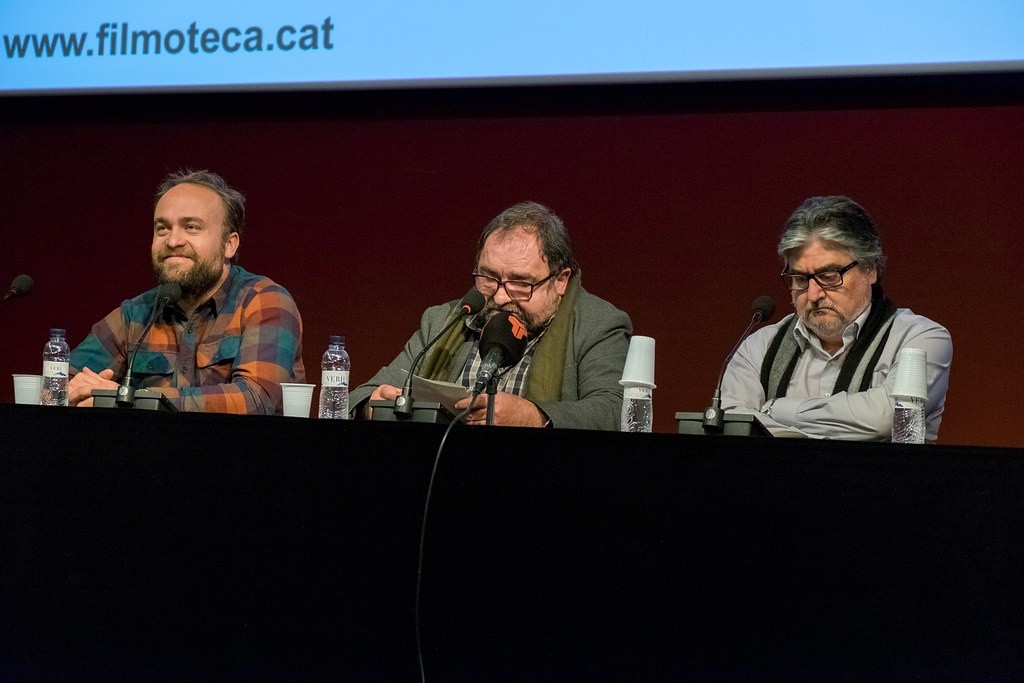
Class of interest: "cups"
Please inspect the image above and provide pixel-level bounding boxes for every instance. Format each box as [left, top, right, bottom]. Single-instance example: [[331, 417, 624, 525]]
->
[[12, 374, 42, 405], [888, 348, 931, 401], [279, 383, 317, 418], [618, 335, 657, 388]]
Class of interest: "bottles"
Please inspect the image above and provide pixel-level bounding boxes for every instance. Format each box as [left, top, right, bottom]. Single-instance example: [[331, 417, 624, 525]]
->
[[40, 328, 70, 407], [620, 384, 653, 433], [318, 335, 351, 419], [891, 396, 925, 444]]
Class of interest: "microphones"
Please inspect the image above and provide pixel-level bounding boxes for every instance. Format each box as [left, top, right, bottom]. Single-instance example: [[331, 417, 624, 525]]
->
[[0, 274, 34, 304], [370, 290, 486, 425], [472, 311, 529, 395], [674, 294, 776, 436], [91, 283, 184, 413]]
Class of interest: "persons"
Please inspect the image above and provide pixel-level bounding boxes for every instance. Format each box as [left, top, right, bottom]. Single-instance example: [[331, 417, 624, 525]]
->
[[721, 197, 954, 443], [349, 202, 633, 431], [42, 169, 306, 416]]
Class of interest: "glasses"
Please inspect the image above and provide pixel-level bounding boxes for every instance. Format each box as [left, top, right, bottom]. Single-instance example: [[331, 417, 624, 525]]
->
[[472, 266, 560, 301], [780, 258, 860, 290]]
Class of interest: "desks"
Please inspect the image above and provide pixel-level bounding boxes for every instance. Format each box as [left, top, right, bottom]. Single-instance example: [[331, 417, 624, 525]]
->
[[0, 406, 1024, 683]]
